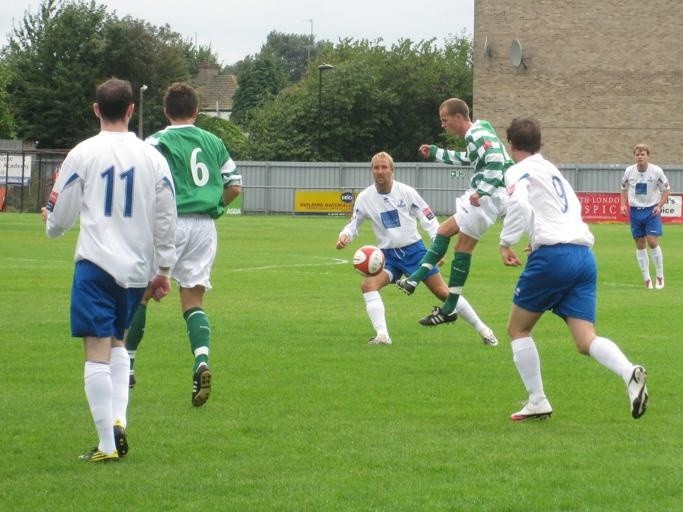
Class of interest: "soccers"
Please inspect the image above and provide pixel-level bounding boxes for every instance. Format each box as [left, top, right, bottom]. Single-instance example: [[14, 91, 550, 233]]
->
[[354, 245, 384, 277]]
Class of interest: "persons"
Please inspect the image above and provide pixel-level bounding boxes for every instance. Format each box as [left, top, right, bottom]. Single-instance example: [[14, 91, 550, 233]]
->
[[337, 157, 498, 348], [498, 115, 648, 422], [41, 80, 177, 463], [127, 82, 240, 409], [621, 145, 671, 290], [395, 97, 514, 328]]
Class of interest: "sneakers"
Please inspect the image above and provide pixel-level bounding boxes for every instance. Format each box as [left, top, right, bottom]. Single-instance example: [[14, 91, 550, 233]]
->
[[509, 398, 552, 421], [367, 337, 392, 345], [643, 278, 653, 289], [655, 276, 664, 290], [627, 364, 649, 419], [192, 365, 213, 407], [395, 280, 417, 296], [78, 447, 119, 462], [418, 306, 457, 327], [479, 326, 498, 347], [113, 421, 128, 456]]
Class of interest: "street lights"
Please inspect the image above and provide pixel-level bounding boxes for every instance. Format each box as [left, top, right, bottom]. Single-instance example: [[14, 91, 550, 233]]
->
[[139, 84, 148, 140], [318, 64, 333, 162]]
